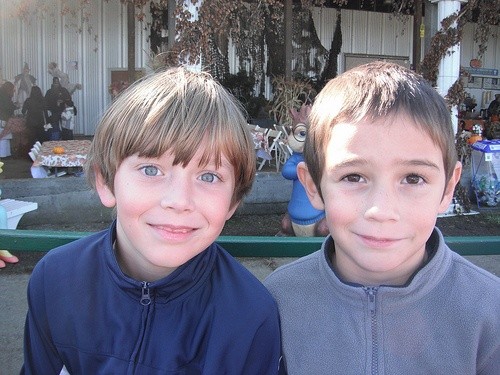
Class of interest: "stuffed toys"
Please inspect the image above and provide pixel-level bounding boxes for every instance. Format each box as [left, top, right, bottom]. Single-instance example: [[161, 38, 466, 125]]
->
[[274, 102, 330, 238]]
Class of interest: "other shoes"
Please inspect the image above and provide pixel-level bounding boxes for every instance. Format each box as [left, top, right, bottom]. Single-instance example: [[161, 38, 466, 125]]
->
[[0, 260, 6, 268], [0, 255, 18, 264]]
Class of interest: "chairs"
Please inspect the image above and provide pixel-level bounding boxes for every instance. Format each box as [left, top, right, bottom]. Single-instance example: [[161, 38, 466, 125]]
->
[[29, 141, 67, 178], [247, 123, 292, 173]]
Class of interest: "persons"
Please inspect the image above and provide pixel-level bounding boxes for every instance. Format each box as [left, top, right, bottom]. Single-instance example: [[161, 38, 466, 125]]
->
[[44, 76, 78, 141], [19, 62, 285, 375], [487, 93, 500, 122], [60, 98, 75, 141], [21, 85, 45, 152], [263, 61, 500, 375], [0, 81, 17, 159]]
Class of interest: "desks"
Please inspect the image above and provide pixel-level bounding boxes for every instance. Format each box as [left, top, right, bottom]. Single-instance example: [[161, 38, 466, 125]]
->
[[38, 140, 91, 179]]
